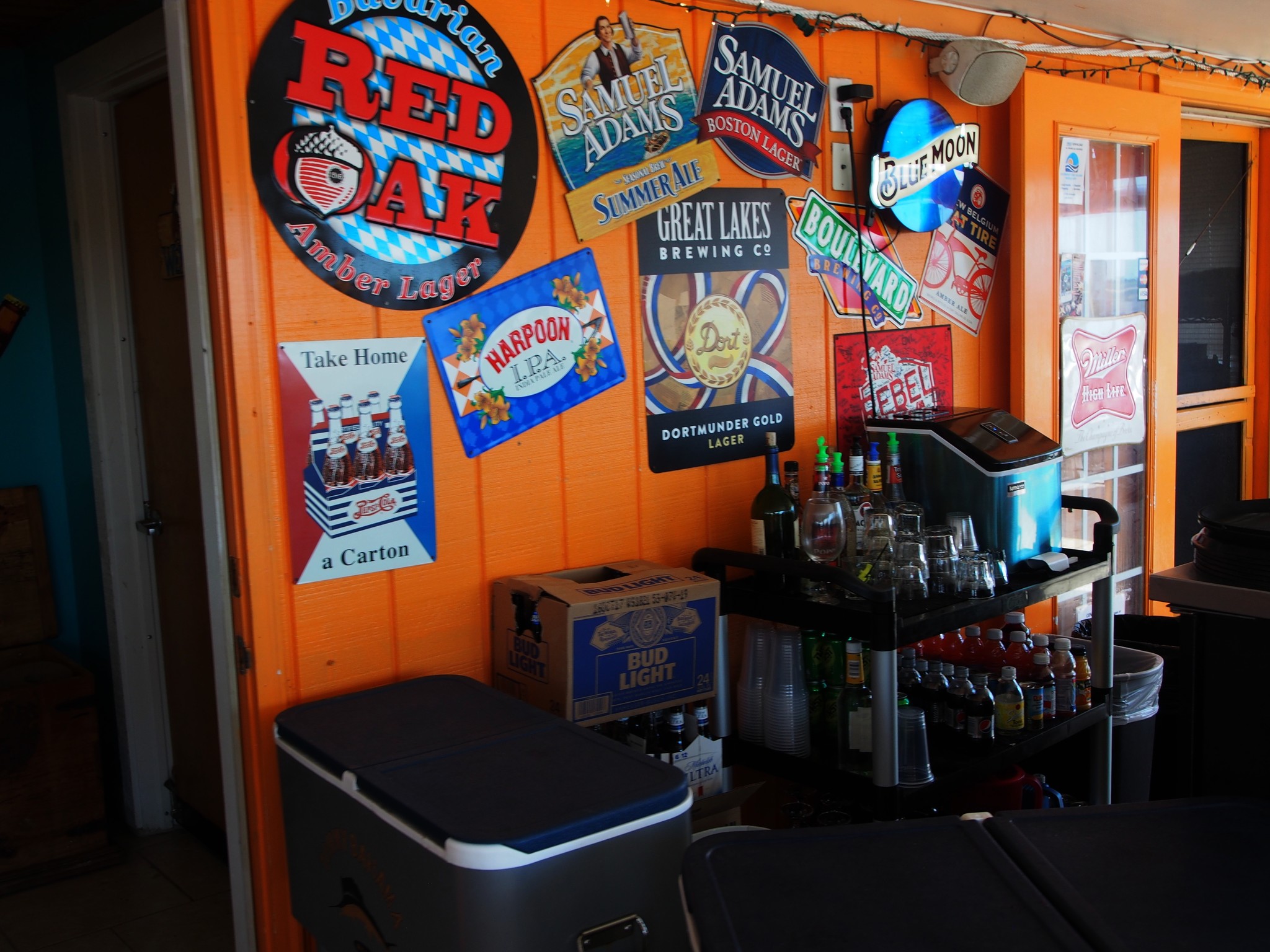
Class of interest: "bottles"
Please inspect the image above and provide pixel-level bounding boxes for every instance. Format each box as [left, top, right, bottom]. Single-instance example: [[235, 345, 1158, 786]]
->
[[811, 437, 857, 591], [994, 666, 1024, 738], [662, 704, 687, 754], [845, 432, 906, 557], [593, 710, 664, 754], [513, 594, 542, 644], [843, 631, 873, 760], [896, 640, 928, 708], [921, 628, 964, 667], [962, 611, 1033, 681], [967, 673, 995, 747], [1033, 774, 1065, 809], [921, 661, 950, 736], [751, 432, 804, 558], [693, 699, 712, 741], [942, 663, 974, 739], [1027, 635, 1091, 718]]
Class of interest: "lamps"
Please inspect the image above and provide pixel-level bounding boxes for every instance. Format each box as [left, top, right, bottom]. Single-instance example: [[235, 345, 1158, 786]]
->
[[928, 40, 1027, 106]]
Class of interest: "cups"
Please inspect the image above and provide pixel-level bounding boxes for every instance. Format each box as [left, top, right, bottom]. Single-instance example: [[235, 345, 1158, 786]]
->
[[736, 620, 777, 746], [925, 525, 960, 604], [897, 706, 935, 789], [857, 502, 931, 611], [944, 511, 1009, 599], [974, 763, 1043, 811], [766, 625, 812, 757]]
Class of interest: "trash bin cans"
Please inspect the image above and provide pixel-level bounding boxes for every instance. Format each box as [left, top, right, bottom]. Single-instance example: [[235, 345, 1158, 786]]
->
[[1074, 638, 1165, 807]]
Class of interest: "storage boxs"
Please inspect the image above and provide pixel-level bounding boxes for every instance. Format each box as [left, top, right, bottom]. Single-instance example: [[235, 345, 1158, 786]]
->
[[493, 558, 722, 726], [274, 675, 694, 952]]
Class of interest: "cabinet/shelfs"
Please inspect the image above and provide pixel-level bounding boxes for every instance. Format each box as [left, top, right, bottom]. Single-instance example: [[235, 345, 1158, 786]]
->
[[690, 495, 1119, 819]]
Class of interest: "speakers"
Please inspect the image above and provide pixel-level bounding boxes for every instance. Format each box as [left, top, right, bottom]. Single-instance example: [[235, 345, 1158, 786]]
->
[[936, 39, 1027, 106]]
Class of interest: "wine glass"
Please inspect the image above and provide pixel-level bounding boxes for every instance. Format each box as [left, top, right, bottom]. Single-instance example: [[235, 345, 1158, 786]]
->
[[800, 498, 846, 604]]
